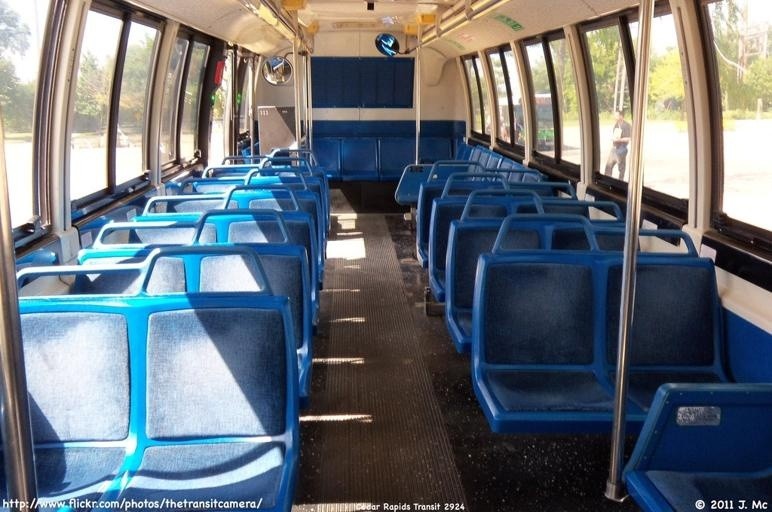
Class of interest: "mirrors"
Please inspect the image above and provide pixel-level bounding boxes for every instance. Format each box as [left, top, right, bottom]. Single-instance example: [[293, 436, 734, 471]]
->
[[374, 32, 400, 56], [259, 55, 293, 86]]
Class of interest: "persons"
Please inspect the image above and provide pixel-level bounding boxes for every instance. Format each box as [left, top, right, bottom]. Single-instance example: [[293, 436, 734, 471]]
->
[[514, 97, 524, 145], [604, 111, 632, 181]]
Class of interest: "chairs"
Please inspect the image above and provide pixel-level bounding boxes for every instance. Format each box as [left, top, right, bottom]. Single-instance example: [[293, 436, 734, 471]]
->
[[1, 147, 332, 512], [237, 130, 457, 180], [395, 141, 772, 512]]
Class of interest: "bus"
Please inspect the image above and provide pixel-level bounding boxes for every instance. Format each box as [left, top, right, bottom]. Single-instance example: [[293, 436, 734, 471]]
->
[[487, 94, 563, 150]]
